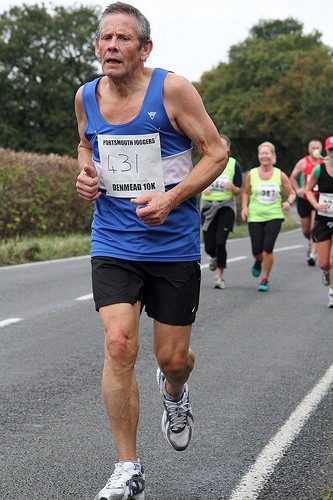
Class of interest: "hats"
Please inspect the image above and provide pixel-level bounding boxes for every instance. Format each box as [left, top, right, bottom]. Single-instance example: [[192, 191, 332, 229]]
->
[[324, 136, 333, 151]]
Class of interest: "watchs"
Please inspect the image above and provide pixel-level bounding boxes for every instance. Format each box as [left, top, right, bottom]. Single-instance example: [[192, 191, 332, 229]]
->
[[286, 200, 293, 207]]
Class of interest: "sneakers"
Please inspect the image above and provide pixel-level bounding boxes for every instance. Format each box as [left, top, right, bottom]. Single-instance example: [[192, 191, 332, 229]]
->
[[156, 364, 193, 452], [94, 458, 145, 500]]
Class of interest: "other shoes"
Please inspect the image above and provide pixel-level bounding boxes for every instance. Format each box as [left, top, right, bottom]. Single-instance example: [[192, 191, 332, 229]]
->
[[321, 269, 329, 285], [251, 258, 263, 277], [257, 279, 269, 292], [306, 253, 316, 267], [213, 278, 226, 288], [327, 290, 333, 308], [209, 256, 217, 270]]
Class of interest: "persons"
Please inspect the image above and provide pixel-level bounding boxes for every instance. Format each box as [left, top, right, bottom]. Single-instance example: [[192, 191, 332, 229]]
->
[[289, 138, 325, 266], [303, 136, 333, 308], [198, 134, 243, 290], [239, 142, 297, 292], [74, 2, 228, 500]]
